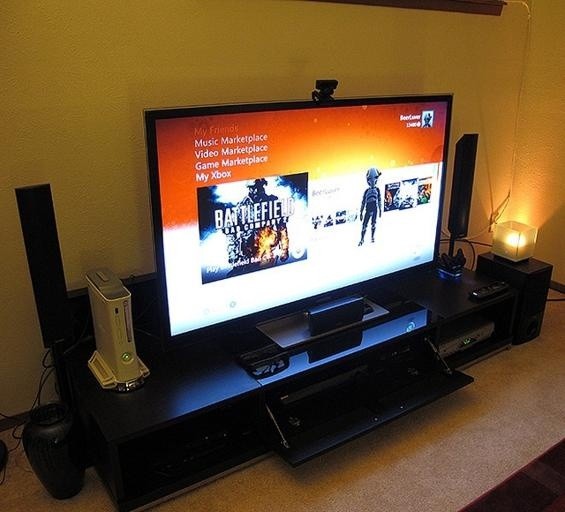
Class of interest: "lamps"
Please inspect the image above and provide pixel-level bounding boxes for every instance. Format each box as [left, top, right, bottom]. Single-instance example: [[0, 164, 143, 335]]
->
[[490, 220, 540, 264]]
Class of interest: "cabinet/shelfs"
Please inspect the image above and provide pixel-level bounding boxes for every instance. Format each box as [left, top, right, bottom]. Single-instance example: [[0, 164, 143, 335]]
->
[[51, 255, 519, 511]]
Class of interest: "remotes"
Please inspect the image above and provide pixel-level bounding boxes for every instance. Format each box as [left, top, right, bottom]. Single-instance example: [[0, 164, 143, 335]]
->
[[468, 280, 510, 302]]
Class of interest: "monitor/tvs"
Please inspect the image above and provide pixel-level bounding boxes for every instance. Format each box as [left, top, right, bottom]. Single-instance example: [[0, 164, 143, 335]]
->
[[142, 93, 454, 353]]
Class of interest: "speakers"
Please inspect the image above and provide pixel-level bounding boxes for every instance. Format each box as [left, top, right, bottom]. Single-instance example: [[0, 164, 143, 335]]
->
[[476, 252, 553, 346], [14, 183, 75, 347], [448, 134, 478, 236]]
